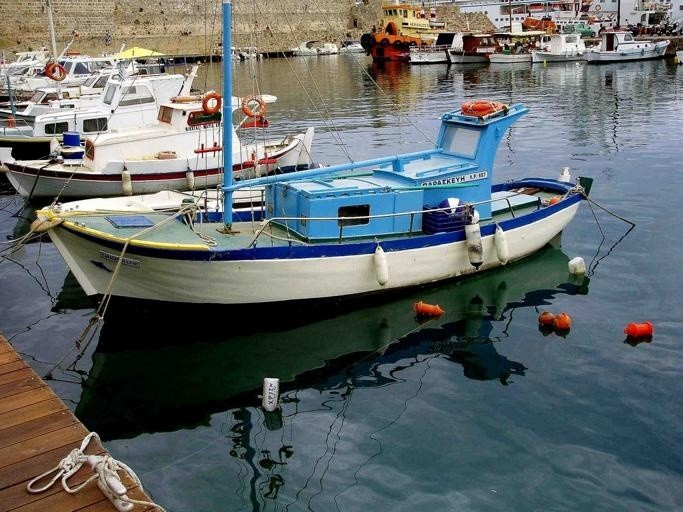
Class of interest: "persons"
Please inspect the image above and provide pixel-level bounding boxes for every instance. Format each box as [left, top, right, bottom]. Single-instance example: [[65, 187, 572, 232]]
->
[[504, 41, 536, 54]]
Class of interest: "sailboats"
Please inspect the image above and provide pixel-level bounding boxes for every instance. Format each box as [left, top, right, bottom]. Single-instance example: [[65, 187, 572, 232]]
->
[[32, 0, 586, 306]]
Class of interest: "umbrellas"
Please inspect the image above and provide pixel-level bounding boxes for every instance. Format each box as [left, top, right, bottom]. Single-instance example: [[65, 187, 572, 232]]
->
[[111, 46, 164, 60]]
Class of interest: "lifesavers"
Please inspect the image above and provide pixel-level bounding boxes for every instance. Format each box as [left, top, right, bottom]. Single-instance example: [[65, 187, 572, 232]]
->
[[461, 99, 488, 112], [49, 62, 66, 81], [241, 94, 266, 118], [202, 91, 221, 113]]
